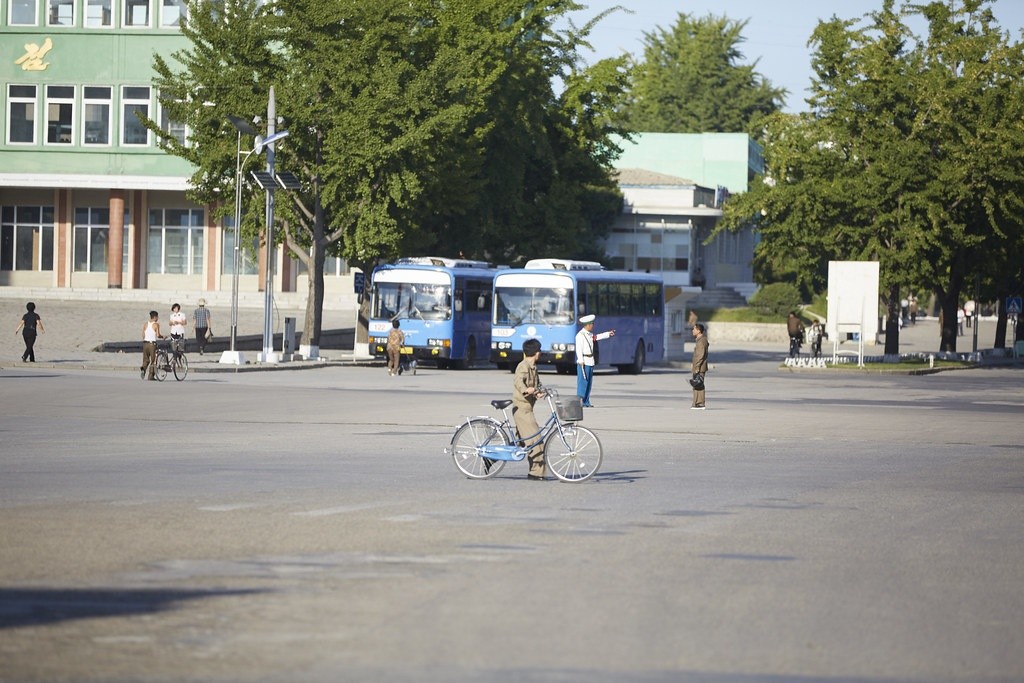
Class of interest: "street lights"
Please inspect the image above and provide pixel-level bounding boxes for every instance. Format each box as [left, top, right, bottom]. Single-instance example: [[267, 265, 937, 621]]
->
[[215, 115, 293, 364]]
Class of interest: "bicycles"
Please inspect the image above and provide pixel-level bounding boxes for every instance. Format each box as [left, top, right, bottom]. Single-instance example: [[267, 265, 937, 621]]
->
[[790, 336, 801, 358], [153, 335, 190, 382], [808, 334, 822, 358], [443, 388, 605, 487]]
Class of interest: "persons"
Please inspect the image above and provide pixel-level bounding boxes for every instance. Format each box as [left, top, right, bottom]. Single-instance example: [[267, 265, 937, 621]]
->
[[16, 302, 44, 363], [140, 311, 163, 380], [689, 310, 697, 328], [939, 309, 943, 336], [901, 297, 915, 324], [481, 339, 546, 478], [169, 303, 186, 357], [386, 320, 405, 376], [192, 298, 211, 355], [692, 324, 709, 410], [575, 314, 615, 409], [964, 301, 974, 327], [808, 319, 822, 354], [787, 312, 805, 356], [957, 305, 964, 336]]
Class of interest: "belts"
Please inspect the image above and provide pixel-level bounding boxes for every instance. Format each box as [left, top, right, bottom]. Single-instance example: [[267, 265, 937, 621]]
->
[[583, 354, 594, 357], [143, 340, 156, 343]]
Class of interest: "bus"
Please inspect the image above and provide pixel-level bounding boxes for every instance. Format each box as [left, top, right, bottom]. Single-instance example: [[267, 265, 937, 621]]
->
[[364, 256, 506, 370], [475, 258, 667, 377]]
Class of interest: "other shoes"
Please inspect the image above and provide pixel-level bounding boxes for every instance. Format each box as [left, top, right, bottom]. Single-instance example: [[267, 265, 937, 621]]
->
[[391, 373, 398, 377], [481, 457, 492, 475], [206, 332, 214, 344], [690, 404, 705, 410], [388, 369, 391, 376], [199, 345, 204, 356], [574, 405, 594, 408], [141, 368, 145, 380], [147, 378, 158, 381], [528, 474, 548, 480], [21, 356, 26, 363], [30, 360, 35, 362]]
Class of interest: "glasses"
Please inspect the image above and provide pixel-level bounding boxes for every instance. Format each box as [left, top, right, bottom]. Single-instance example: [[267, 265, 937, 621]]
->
[[591, 323, 595, 325]]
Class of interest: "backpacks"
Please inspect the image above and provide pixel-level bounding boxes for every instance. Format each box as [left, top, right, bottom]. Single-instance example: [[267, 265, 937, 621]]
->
[[689, 373, 705, 391]]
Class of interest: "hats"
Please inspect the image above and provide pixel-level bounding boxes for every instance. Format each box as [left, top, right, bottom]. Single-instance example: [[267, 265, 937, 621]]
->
[[578, 314, 596, 324], [197, 297, 207, 305]]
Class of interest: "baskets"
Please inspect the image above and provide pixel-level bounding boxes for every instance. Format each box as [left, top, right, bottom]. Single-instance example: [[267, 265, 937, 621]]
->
[[554, 397, 584, 422], [171, 339, 185, 352]]
